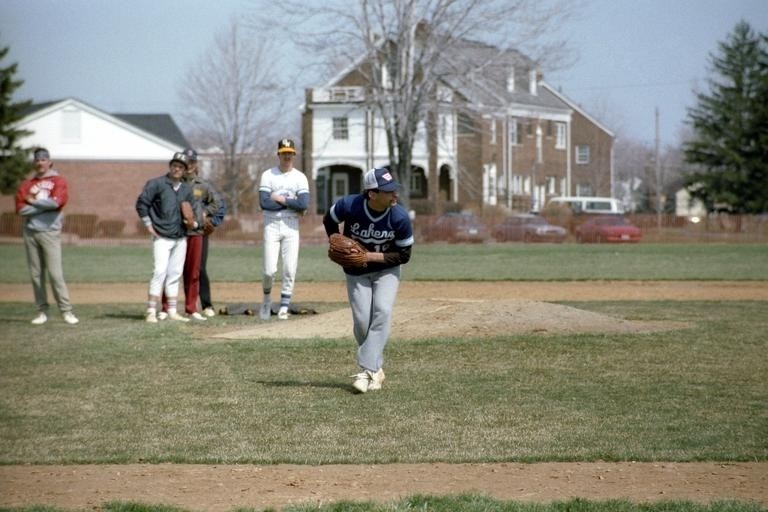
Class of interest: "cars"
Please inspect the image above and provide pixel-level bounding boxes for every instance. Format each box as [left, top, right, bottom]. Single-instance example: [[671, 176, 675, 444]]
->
[[491, 214, 568, 244], [573, 216, 642, 244], [420, 212, 488, 242]]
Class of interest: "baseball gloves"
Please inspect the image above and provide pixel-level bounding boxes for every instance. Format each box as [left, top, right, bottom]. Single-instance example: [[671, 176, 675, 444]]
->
[[328, 233, 368, 267]]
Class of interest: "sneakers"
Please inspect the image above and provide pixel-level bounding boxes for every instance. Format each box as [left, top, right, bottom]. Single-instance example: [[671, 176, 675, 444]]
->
[[260, 303, 270, 320], [349, 373, 369, 393], [62, 311, 79, 324], [277, 307, 288, 319], [32, 312, 47, 324], [147, 307, 215, 322], [365, 369, 385, 391]]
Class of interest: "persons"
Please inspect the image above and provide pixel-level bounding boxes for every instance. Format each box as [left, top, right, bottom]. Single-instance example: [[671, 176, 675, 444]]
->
[[135, 151, 196, 323], [15, 148, 79, 325], [259, 138, 311, 320], [182, 165, 227, 318], [322, 168, 414, 393], [156, 148, 207, 321]]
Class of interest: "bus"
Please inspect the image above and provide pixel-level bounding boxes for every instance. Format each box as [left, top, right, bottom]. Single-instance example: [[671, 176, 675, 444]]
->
[[546, 196, 625, 217]]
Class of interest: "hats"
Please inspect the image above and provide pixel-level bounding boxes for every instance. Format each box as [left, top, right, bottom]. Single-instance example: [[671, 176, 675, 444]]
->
[[278, 139, 297, 153], [170, 152, 190, 170], [182, 149, 197, 161], [363, 168, 401, 191]]
